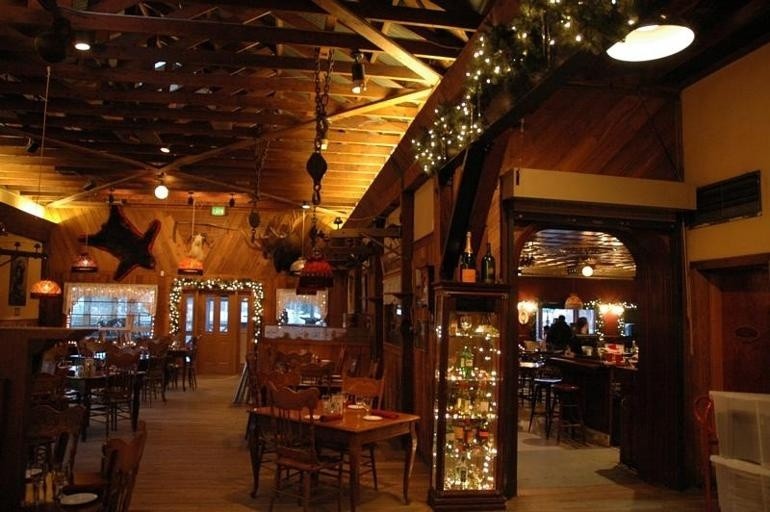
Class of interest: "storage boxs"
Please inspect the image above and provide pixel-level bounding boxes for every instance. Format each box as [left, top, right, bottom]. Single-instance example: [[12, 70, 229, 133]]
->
[[706, 387, 770, 471], [708, 452, 770, 512]]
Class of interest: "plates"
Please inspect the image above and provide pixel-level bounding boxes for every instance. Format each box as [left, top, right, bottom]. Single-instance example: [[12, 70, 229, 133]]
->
[[60, 492, 97, 505], [363, 415, 381, 421]]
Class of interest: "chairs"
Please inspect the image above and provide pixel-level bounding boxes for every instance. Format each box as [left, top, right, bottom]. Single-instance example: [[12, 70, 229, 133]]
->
[[228, 329, 422, 512], [1, 319, 202, 511]]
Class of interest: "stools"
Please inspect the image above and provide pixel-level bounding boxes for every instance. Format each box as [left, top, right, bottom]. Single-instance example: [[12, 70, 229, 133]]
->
[[516, 334, 641, 448]]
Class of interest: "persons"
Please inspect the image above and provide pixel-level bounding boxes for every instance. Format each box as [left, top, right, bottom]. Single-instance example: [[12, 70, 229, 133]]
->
[[542, 314, 590, 353], [33, 337, 63, 376]]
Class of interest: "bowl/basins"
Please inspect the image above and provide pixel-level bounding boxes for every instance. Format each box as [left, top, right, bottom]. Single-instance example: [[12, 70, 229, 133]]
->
[[301, 317, 321, 324]]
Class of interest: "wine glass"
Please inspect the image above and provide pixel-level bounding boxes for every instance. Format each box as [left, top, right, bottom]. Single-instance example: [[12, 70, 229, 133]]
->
[[24, 457, 70, 508], [305, 391, 365, 420]]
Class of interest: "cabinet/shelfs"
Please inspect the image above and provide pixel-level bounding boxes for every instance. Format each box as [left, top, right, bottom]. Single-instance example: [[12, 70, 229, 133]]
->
[[426, 279, 518, 509]]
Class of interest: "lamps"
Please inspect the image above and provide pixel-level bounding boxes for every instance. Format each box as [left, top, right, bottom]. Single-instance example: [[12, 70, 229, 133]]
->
[[69, 196, 98, 273], [347, 45, 368, 97], [288, 200, 337, 297], [28, 257, 64, 301], [175, 201, 209, 277], [602, 0, 698, 65]]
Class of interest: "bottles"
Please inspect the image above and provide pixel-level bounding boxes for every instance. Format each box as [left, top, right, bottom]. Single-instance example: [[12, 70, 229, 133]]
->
[[480, 243, 495, 285], [446, 316, 499, 490], [580, 339, 638, 366], [460, 231, 476, 282]]
